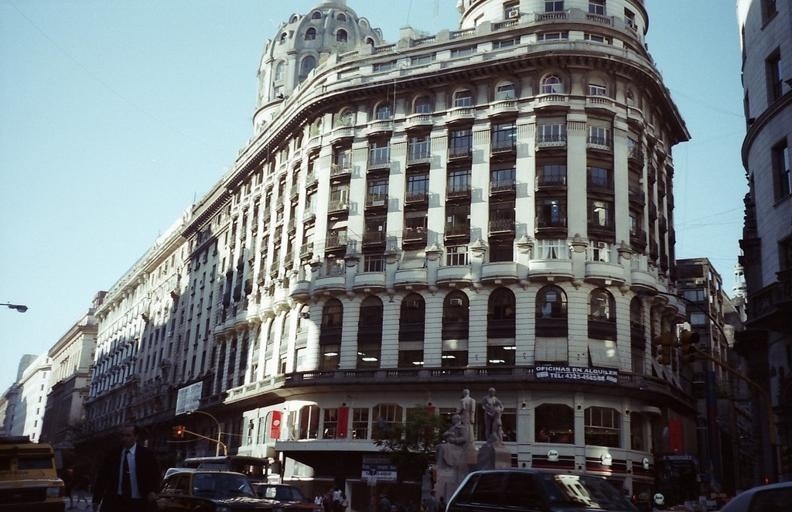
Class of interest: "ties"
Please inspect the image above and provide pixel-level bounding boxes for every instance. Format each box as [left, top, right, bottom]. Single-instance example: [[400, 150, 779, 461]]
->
[[122, 447, 132, 499]]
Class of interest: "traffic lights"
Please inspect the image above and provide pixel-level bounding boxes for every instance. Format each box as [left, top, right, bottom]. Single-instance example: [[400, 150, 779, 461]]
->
[[176, 426, 185, 439], [270, 410, 282, 439], [680, 329, 700, 362]]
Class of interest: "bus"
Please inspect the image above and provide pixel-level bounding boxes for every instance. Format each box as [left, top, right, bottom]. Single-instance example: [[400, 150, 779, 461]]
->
[[183, 456, 269, 483]]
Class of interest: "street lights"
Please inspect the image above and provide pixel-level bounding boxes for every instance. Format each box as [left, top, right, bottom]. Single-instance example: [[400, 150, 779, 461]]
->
[[185, 410, 221, 457], [638, 289, 743, 490], [0, 304, 28, 312]]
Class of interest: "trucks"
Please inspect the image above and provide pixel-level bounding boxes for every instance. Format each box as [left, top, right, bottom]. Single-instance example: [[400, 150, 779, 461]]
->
[[0, 440, 65, 512]]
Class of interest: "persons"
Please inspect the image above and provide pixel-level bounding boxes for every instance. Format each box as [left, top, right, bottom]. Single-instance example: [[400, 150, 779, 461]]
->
[[482, 387, 504, 441], [437, 389, 476, 467], [92, 424, 161, 512], [314, 496, 323, 512], [333, 489, 342, 502]]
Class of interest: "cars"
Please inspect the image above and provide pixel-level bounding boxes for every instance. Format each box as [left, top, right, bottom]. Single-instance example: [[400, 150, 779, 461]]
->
[[715, 481, 792, 511], [144, 468, 321, 512], [445, 467, 641, 512]]
